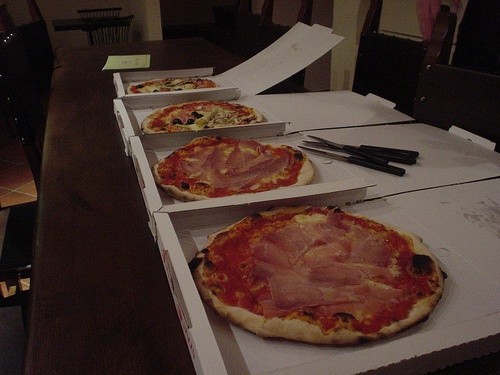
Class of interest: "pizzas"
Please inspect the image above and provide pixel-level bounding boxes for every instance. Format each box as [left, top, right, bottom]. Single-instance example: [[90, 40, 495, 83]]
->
[[140, 101, 264, 134], [126, 77, 218, 94], [187, 206, 448, 347], [152, 135, 314, 200]]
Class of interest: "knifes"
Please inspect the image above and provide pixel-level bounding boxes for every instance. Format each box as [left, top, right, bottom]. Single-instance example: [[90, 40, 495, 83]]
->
[[297, 134, 421, 177]]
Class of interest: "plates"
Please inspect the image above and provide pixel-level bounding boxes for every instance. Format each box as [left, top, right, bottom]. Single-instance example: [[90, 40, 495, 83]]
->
[[101, 54, 151, 71]]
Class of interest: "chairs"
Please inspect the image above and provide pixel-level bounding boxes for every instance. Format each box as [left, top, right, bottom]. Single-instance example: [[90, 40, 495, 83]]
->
[[77, 7, 134, 45], [412, 64, 499, 144], [351, 0, 457, 127], [230, 0, 314, 88], [0, 130, 39, 306]]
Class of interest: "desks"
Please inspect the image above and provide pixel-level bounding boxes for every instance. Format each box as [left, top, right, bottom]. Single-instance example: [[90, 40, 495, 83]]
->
[[53, 15, 134, 31], [26, 36, 500, 375]]
